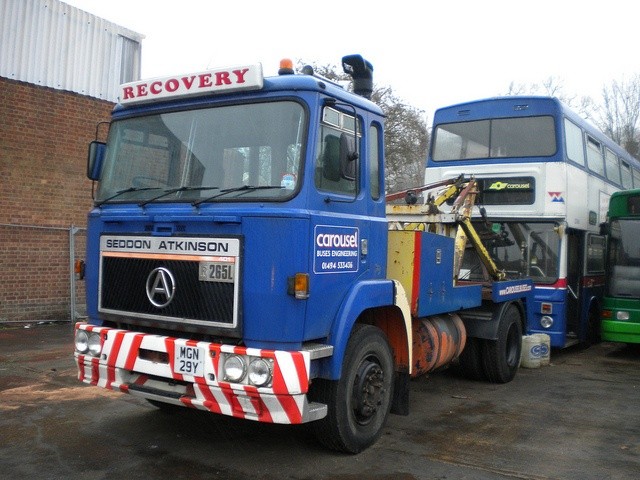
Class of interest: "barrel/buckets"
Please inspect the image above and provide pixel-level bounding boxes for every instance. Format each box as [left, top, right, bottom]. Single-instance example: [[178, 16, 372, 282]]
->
[[533, 333, 551, 365], [522, 336, 540, 368]]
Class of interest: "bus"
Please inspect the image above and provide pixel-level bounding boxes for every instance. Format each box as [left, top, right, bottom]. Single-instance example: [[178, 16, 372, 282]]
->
[[425, 96, 639, 348], [601, 189, 640, 342]]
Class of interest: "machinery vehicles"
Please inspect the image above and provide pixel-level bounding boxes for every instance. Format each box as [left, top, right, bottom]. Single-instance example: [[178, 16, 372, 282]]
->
[[74, 54, 534, 454]]
